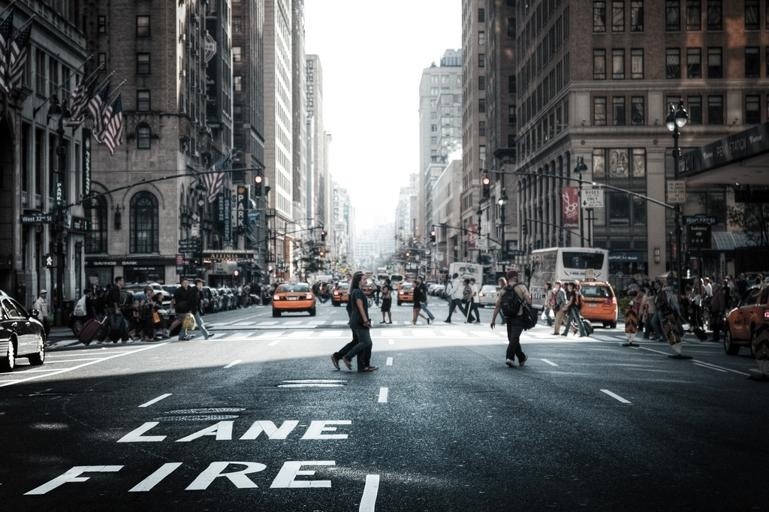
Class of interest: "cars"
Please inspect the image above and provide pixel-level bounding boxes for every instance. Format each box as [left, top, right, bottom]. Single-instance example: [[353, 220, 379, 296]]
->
[[272, 281, 316, 316], [0, 287, 46, 368], [313, 267, 446, 306], [572, 280, 619, 329], [71, 282, 271, 335], [479, 284, 500, 308]]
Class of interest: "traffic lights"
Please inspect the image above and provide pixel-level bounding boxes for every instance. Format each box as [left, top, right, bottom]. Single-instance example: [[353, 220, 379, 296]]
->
[[429, 230, 436, 242], [255, 174, 263, 197], [321, 231, 326, 241], [482, 175, 491, 198], [263, 184, 271, 196]]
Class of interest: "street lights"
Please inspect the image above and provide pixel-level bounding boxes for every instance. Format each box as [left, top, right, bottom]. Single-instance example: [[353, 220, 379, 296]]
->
[[498, 186, 509, 272], [664, 96, 692, 319]]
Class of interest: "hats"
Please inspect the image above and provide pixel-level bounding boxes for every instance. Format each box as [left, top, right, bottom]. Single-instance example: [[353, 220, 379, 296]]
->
[[40, 289, 48, 294], [506, 270, 518, 280]]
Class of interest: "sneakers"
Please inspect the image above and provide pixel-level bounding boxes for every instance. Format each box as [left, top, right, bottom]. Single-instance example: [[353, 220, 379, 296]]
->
[[504, 352, 529, 368], [330, 353, 378, 372], [380, 315, 481, 324], [553, 328, 589, 337]]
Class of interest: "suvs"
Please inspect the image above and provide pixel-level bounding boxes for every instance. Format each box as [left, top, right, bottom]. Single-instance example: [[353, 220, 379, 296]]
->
[[722, 282, 769, 361]]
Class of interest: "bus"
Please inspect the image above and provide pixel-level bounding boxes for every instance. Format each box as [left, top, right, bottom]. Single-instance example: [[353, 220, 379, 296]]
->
[[445, 262, 483, 298], [524, 246, 610, 312]]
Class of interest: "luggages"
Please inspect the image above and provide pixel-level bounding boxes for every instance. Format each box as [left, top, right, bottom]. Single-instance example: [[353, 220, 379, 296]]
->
[[77, 319, 101, 344], [583, 319, 594, 335]]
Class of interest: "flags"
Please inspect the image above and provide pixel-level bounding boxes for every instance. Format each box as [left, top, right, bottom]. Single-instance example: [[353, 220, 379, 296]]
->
[[66, 65, 123, 155], [204, 157, 224, 204], [0, 12, 12, 92], [12, 23, 31, 93]]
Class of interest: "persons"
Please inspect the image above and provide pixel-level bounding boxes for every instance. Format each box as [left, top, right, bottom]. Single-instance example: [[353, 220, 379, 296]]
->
[[489, 271, 532, 368], [33, 275, 214, 342], [332, 273, 372, 369], [344, 274, 379, 372], [382, 274, 767, 341]]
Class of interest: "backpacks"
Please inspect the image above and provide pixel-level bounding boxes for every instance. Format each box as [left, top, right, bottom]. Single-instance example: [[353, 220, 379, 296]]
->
[[500, 289, 521, 317]]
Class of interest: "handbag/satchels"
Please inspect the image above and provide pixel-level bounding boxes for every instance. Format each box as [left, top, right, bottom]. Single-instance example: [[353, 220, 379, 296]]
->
[[522, 303, 538, 330]]
